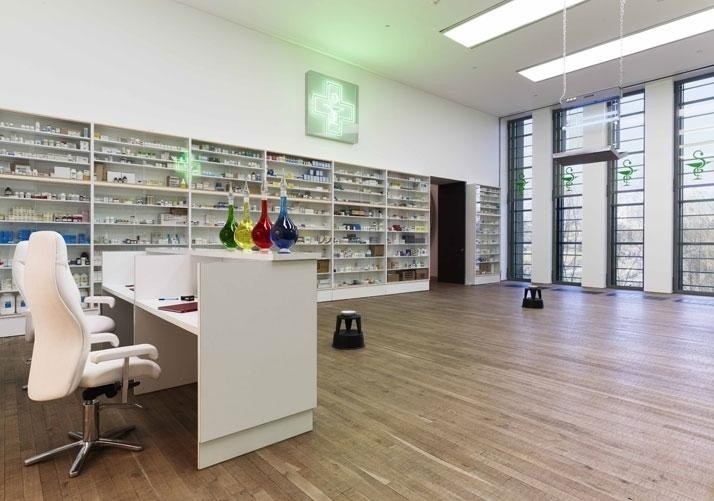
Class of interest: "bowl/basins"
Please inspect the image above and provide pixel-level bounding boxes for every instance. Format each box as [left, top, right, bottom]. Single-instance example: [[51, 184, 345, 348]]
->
[[529, 285, 538, 288], [340, 309, 357, 317]]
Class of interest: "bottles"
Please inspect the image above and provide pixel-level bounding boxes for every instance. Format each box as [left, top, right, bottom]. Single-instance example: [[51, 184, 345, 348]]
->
[[219, 197, 243, 252], [270, 187, 302, 254], [0, 115, 192, 290], [331, 236, 432, 290], [252, 192, 277, 253], [186, 137, 426, 232], [233, 191, 258, 250]]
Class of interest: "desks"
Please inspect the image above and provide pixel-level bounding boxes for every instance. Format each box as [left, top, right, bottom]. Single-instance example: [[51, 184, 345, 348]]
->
[[100, 245, 323, 471]]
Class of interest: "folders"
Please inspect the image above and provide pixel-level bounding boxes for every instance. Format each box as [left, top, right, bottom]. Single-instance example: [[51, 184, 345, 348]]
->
[[159, 302, 197, 313]]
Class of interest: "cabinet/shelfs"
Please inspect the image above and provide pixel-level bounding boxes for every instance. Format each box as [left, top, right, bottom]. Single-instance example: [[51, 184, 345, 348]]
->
[[333, 160, 387, 300], [190, 137, 265, 249], [386, 169, 430, 294], [91, 122, 190, 313], [466, 182, 501, 285], [266, 150, 333, 303], [0, 105, 92, 338]]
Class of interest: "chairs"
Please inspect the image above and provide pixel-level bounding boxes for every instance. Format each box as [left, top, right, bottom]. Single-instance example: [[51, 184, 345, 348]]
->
[[22, 229, 161, 478], [9, 240, 116, 390]]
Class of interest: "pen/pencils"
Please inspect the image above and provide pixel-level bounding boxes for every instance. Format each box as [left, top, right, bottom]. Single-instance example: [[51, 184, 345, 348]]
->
[[159, 298, 179, 300]]
